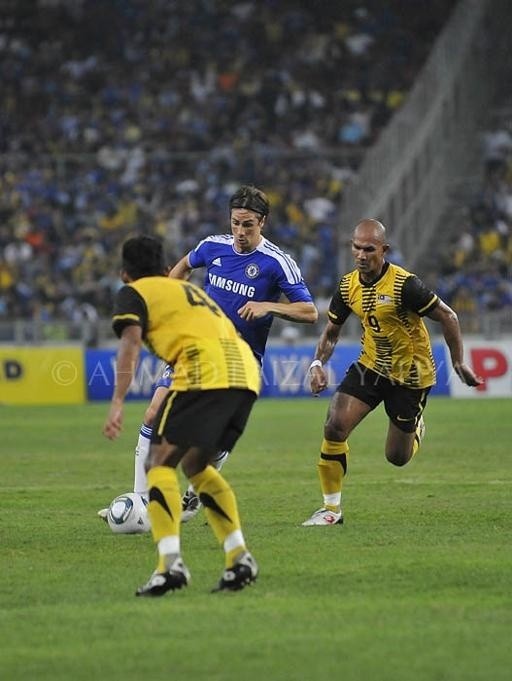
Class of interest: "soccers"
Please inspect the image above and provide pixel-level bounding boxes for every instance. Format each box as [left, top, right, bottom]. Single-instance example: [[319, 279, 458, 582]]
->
[[107, 493, 150, 533]]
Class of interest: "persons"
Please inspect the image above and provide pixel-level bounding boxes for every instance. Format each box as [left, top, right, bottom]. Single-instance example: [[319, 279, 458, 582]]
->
[[302, 219, 485, 526], [102, 237, 264, 597], [97, 187, 319, 524]]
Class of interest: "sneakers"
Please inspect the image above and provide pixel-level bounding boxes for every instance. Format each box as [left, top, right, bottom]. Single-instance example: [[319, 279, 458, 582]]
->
[[301, 507, 343, 527], [210, 550, 258, 593], [419, 415, 425, 438], [97, 508, 110, 520], [181, 489, 202, 522], [136, 556, 190, 597]]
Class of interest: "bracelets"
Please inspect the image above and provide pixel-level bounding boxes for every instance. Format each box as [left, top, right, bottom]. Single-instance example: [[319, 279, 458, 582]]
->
[[309, 360, 323, 372]]
